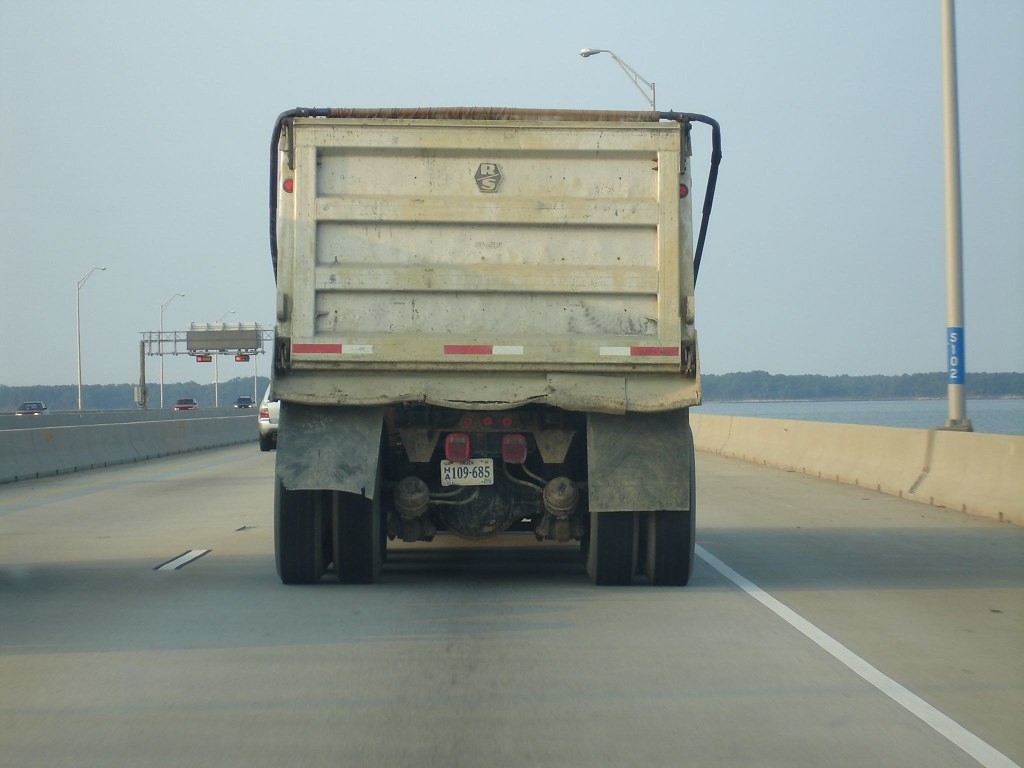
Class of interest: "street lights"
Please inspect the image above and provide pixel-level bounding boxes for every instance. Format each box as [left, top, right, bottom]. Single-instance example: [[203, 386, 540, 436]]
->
[[77, 268, 107, 410], [160, 293, 186, 408], [580, 47, 658, 111], [215, 310, 236, 408]]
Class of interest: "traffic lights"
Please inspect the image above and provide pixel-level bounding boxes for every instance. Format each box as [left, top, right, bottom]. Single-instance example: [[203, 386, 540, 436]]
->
[[235, 355, 249, 361], [196, 355, 212, 362]]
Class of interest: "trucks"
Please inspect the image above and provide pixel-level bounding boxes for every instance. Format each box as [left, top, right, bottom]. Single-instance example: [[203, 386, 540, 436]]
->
[[270, 105, 721, 586]]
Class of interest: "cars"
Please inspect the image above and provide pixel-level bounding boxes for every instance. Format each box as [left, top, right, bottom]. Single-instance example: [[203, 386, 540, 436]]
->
[[233, 395, 255, 408], [258, 381, 281, 451], [15, 401, 49, 416], [174, 398, 198, 411]]
